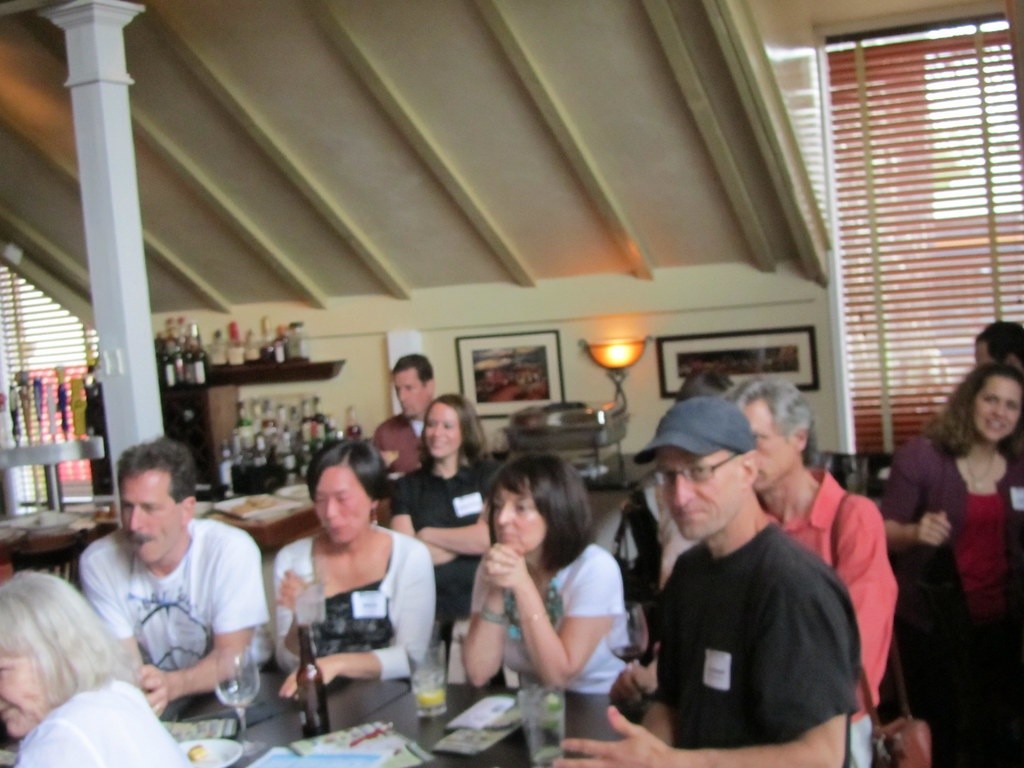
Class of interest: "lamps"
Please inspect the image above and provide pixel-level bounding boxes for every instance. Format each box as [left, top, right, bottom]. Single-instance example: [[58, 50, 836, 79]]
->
[[583, 330, 652, 406]]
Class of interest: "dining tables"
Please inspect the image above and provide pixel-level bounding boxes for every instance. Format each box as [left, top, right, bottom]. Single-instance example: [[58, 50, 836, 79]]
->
[[178, 668, 648, 768]]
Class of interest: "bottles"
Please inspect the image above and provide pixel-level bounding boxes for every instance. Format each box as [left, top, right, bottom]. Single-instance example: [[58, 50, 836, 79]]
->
[[153, 314, 362, 493], [296, 627, 330, 740]]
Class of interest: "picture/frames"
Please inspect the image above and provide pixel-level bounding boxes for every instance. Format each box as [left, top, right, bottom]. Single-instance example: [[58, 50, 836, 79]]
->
[[656, 322, 822, 404], [446, 327, 571, 420]]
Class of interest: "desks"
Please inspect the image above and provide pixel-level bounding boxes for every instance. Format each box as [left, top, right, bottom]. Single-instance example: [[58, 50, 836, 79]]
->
[[486, 452, 665, 494]]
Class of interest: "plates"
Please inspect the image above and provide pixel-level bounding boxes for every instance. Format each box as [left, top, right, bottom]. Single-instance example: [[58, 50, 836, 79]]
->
[[213, 494, 305, 520], [96, 517, 121, 526], [275, 483, 311, 502], [0, 508, 81, 544], [178, 738, 243, 768]]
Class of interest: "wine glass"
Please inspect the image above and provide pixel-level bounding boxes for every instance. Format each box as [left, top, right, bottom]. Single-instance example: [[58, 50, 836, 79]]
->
[[214, 642, 266, 759], [606, 601, 654, 713]]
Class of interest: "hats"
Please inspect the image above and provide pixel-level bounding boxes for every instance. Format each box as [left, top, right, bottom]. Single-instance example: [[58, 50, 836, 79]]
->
[[634, 396, 756, 465]]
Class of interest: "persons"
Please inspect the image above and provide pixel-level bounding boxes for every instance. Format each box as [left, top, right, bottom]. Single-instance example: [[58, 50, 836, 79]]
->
[[721, 376, 900, 768], [654, 362, 733, 591], [458, 451, 630, 694], [370, 354, 436, 474], [390, 393, 502, 620], [0, 569, 192, 768], [553, 395, 861, 768], [272, 438, 436, 699], [78, 440, 271, 717], [974, 321, 1024, 371], [879, 362, 1024, 768]]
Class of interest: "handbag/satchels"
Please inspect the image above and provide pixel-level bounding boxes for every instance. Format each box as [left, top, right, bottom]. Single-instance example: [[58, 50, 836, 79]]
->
[[875, 717, 932, 768]]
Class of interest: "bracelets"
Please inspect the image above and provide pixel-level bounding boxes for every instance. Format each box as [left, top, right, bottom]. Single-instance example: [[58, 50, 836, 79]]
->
[[519, 612, 547, 623], [480, 606, 507, 624]]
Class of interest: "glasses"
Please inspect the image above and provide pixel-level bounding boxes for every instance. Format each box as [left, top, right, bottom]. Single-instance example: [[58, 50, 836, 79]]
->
[[654, 452, 742, 487]]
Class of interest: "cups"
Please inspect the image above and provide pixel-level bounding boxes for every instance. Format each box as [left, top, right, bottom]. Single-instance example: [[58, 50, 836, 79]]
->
[[405, 640, 446, 718], [516, 683, 567, 768], [291, 571, 330, 626]]
